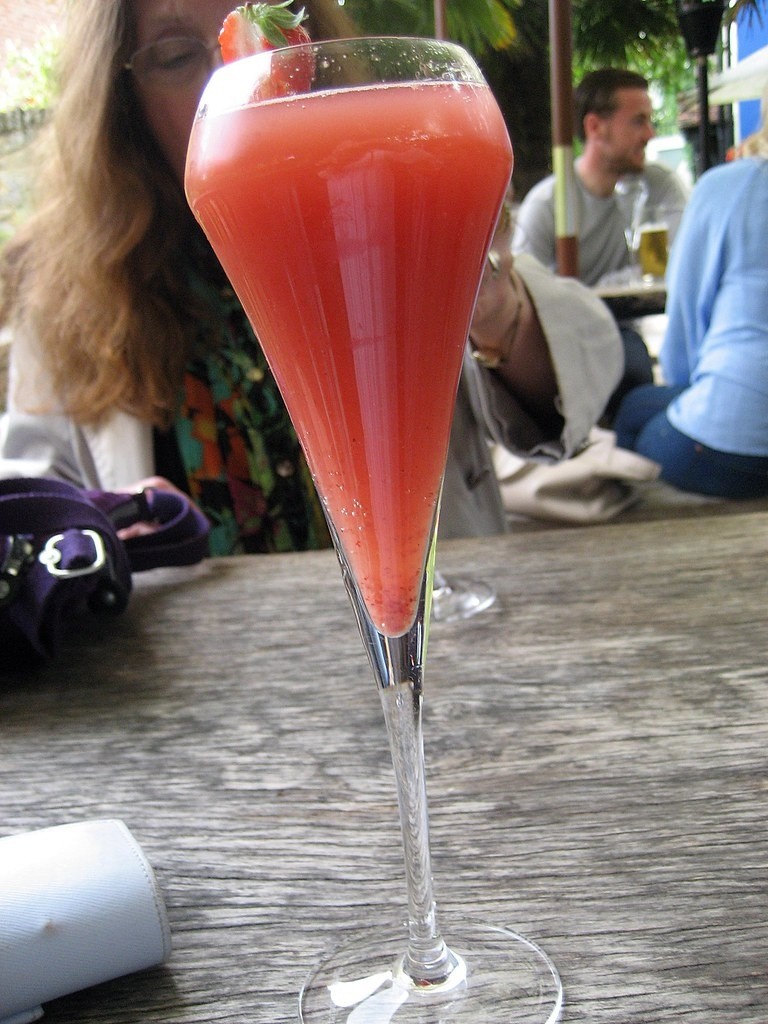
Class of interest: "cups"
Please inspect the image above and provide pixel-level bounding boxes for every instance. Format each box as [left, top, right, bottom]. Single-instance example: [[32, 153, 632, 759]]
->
[[639, 225, 668, 286]]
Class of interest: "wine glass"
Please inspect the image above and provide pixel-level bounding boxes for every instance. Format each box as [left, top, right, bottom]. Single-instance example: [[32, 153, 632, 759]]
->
[[612, 174, 648, 286], [182, 36, 563, 1024], [429, 569, 495, 627]]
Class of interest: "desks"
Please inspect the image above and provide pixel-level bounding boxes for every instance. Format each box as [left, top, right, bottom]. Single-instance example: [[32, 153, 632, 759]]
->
[[1, 512, 768, 1024]]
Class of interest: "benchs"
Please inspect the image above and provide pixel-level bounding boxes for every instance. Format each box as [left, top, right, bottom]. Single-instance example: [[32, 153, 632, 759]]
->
[[506, 471, 768, 529]]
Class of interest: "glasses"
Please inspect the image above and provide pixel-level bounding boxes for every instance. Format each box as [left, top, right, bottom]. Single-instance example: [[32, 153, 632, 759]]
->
[[125, 36, 219, 88]]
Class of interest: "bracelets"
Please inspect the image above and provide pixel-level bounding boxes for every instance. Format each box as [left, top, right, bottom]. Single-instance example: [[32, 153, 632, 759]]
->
[[466, 276, 524, 370]]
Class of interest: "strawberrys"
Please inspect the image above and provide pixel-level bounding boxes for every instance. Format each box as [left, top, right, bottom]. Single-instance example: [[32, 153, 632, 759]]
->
[[218, 0, 319, 105]]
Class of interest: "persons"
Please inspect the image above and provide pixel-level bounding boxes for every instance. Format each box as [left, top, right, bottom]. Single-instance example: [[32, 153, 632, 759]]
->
[[614, 154, 767, 505], [0, 0, 627, 557], [512, 67, 693, 431]]
